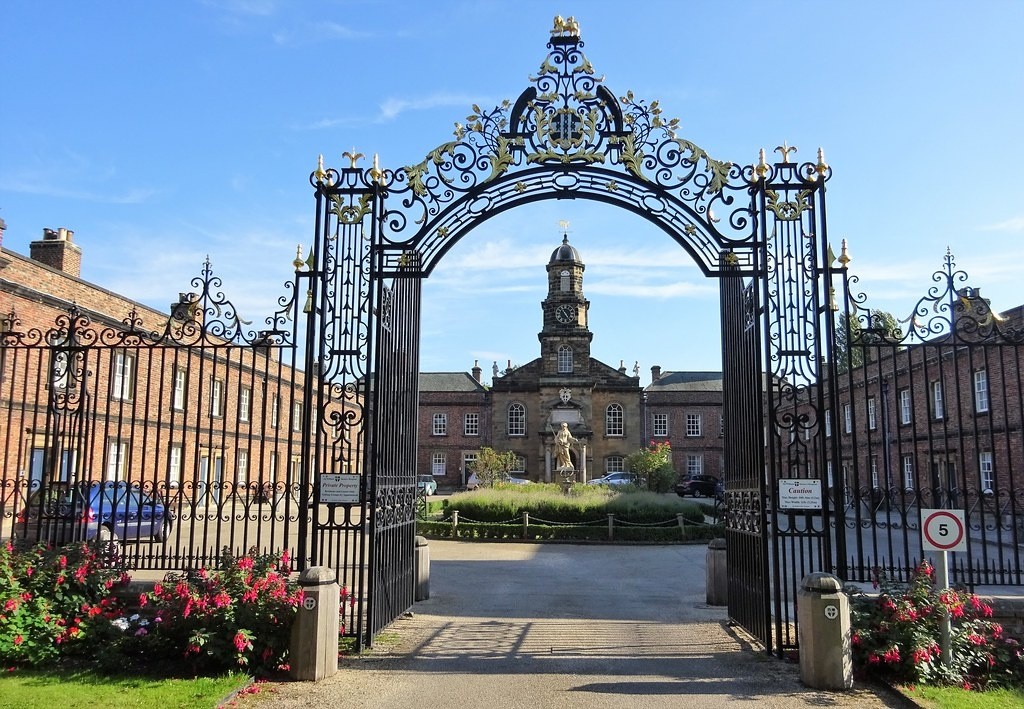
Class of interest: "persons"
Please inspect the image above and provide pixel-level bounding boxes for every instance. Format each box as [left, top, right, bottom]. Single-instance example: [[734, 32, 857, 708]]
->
[[492, 361, 499, 377], [555, 421, 578, 471], [633, 361, 640, 376]]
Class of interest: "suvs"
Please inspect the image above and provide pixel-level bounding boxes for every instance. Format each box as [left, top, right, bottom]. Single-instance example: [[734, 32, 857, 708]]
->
[[467, 469, 533, 491], [18, 481, 175, 554], [676, 474, 719, 498]]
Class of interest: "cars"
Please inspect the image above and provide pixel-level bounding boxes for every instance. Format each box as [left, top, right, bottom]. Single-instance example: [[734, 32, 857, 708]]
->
[[416, 474, 437, 496], [589, 472, 647, 489]]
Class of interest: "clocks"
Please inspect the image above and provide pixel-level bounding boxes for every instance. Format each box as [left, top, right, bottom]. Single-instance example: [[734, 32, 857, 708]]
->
[[555, 305, 575, 324]]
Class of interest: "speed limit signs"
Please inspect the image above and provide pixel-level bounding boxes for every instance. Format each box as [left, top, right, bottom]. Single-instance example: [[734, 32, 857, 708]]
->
[[920, 509, 966, 552]]
